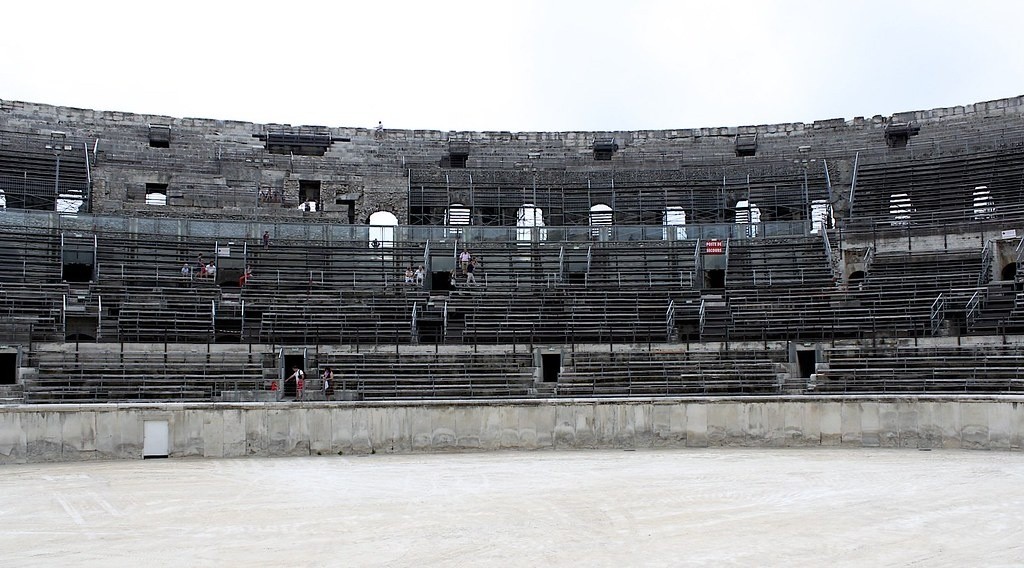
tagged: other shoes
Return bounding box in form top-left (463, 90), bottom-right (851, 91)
top-left (292, 399), bottom-right (297, 402)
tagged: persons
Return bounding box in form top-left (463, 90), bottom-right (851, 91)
top-left (239, 264), bottom-right (254, 287)
top-left (459, 248), bottom-right (482, 287)
top-left (322, 367), bottom-right (334, 401)
top-left (262, 230), bottom-right (270, 249)
top-left (305, 196), bottom-right (319, 211)
top-left (0, 195), bottom-right (6, 210)
top-left (285, 365), bottom-right (306, 402)
top-left (405, 266), bottom-right (425, 287)
top-left (374, 121), bottom-right (385, 140)
top-left (371, 239), bottom-right (381, 248)
top-left (271, 380), bottom-right (279, 392)
top-left (181, 252), bottom-right (215, 282)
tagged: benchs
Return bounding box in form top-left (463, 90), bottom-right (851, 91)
top-left (0, 121), bottom-right (1024, 410)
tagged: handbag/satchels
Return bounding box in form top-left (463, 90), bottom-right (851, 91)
top-left (324, 381), bottom-right (329, 390)
